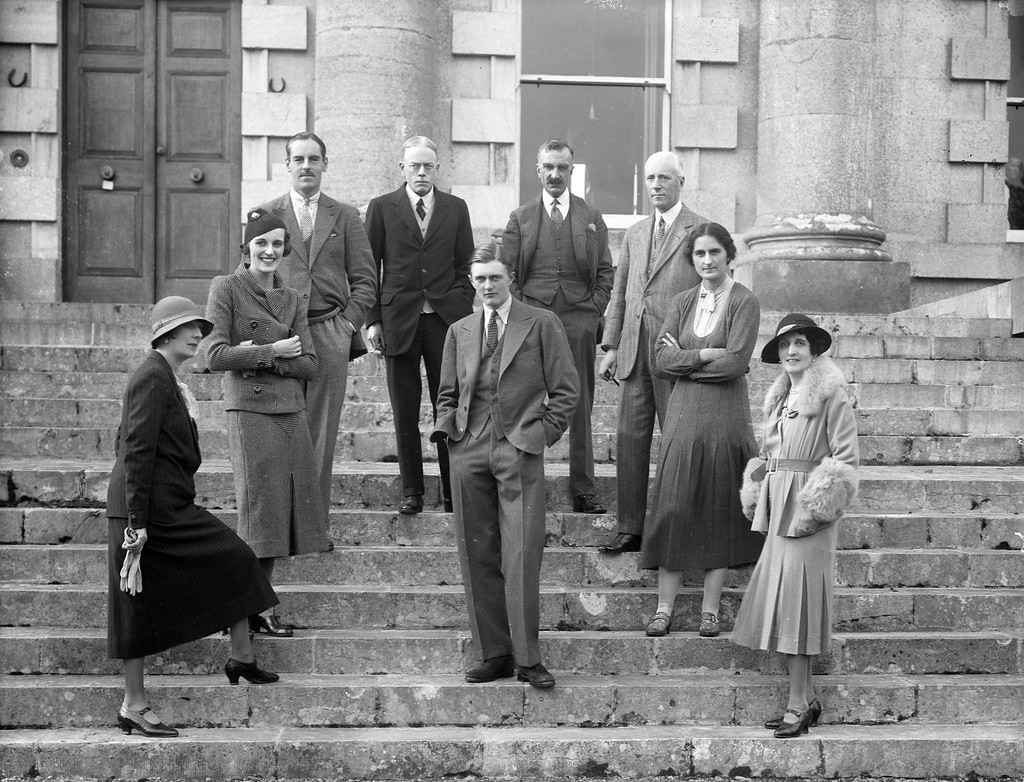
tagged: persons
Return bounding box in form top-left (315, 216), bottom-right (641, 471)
top-left (259, 132), bottom-right (378, 553)
top-left (365, 136), bottom-right (475, 513)
top-left (741, 312), bottom-right (860, 740)
top-left (597, 150), bottom-right (713, 554)
top-left (436, 244), bottom-right (580, 688)
top-left (205, 208), bottom-right (329, 638)
top-left (504, 139), bottom-right (614, 514)
top-left (648, 221), bottom-right (761, 638)
top-left (106, 295), bottom-right (279, 737)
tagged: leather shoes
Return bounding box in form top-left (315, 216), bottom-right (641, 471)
top-left (444, 499), bottom-right (453, 512)
top-left (398, 494), bottom-right (424, 514)
top-left (597, 532), bottom-right (642, 554)
top-left (574, 494), bottom-right (607, 514)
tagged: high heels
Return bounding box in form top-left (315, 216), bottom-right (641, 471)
top-left (765, 696), bottom-right (822, 729)
top-left (225, 657), bottom-right (279, 685)
top-left (117, 705), bottom-right (179, 737)
top-left (248, 612), bottom-right (294, 636)
top-left (646, 608), bottom-right (674, 636)
top-left (698, 612), bottom-right (721, 636)
top-left (774, 708), bottom-right (812, 736)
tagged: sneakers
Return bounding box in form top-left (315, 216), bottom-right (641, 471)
top-left (465, 653), bottom-right (515, 682)
top-left (518, 662), bottom-right (555, 688)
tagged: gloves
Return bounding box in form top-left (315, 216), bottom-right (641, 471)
top-left (118, 526), bottom-right (144, 595)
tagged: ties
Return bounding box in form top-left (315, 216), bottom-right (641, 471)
top-left (488, 310), bottom-right (499, 353)
top-left (551, 198), bottom-right (564, 231)
top-left (416, 199), bottom-right (426, 221)
top-left (301, 198), bottom-right (314, 242)
top-left (654, 215), bottom-right (665, 249)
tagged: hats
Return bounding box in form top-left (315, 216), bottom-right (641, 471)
top-left (761, 313), bottom-right (832, 363)
top-left (244, 208), bottom-right (286, 246)
top-left (150, 296), bottom-right (214, 347)
